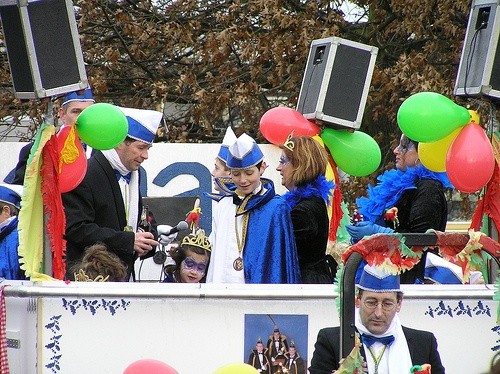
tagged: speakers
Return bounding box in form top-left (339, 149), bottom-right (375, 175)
top-left (296, 37), bottom-right (379, 132)
top-left (1, 0), bottom-right (89, 100)
top-left (452, 1), bottom-right (500, 100)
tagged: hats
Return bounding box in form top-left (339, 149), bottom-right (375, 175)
top-left (357, 261), bottom-right (401, 293)
top-left (218, 126), bottom-right (237, 163)
top-left (0, 179), bottom-right (23, 210)
top-left (227, 132), bottom-right (265, 170)
top-left (61, 82), bottom-right (96, 107)
top-left (117, 106), bottom-right (164, 145)
top-left (425, 251), bottom-right (463, 284)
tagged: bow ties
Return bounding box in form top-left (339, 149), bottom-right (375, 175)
top-left (362, 333), bottom-right (395, 347)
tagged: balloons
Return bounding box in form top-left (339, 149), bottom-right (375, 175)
top-left (304, 133), bottom-right (336, 201)
top-left (396, 92), bottom-right (470, 144)
top-left (74, 102), bottom-right (129, 150)
top-left (446, 119), bottom-right (496, 194)
top-left (47, 123), bottom-right (87, 194)
top-left (259, 107), bottom-right (322, 147)
top-left (320, 127), bottom-right (382, 177)
top-left (418, 110), bottom-right (480, 173)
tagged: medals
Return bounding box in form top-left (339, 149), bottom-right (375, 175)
top-left (123, 224), bottom-right (134, 234)
top-left (232, 256), bottom-right (244, 270)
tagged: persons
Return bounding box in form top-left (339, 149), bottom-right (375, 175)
top-left (61, 244), bottom-right (128, 286)
top-left (1, 177), bottom-right (43, 283)
top-left (273, 129), bottom-right (336, 284)
top-left (13, 82), bottom-right (101, 191)
top-left (305, 263), bottom-right (446, 374)
top-left (344, 130), bottom-right (449, 285)
top-left (203, 124), bottom-right (245, 284)
top-left (56, 110), bottom-right (159, 283)
top-left (159, 231), bottom-right (213, 285)
top-left (247, 324), bottom-right (305, 374)
top-left (212, 133), bottom-right (301, 284)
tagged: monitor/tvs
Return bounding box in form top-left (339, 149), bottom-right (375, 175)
top-left (143, 196), bottom-right (199, 240)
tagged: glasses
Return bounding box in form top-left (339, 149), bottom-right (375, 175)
top-left (357, 297), bottom-right (399, 311)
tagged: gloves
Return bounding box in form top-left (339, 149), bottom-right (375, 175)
top-left (157, 225), bottom-right (178, 246)
top-left (344, 221), bottom-right (393, 242)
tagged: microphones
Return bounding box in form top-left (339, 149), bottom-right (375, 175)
top-left (157, 220), bottom-right (188, 237)
